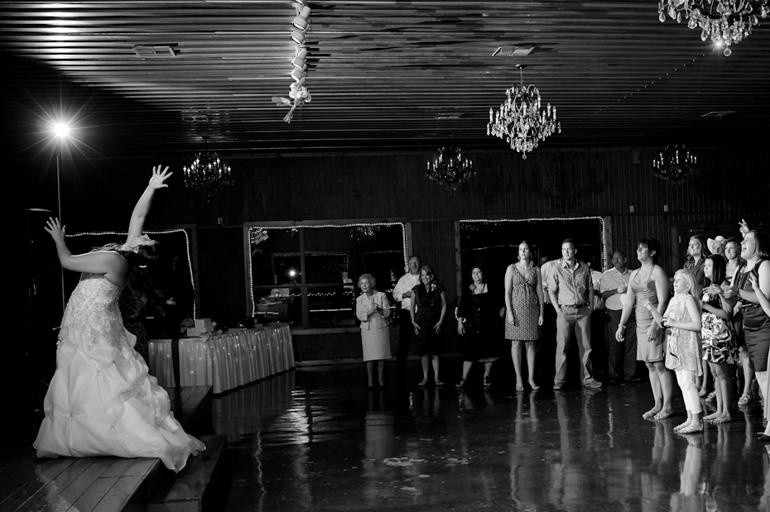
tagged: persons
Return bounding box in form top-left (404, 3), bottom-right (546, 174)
top-left (356, 255), bottom-right (447, 387)
top-left (32, 163), bottom-right (208, 474)
top-left (454, 219), bottom-right (770, 442)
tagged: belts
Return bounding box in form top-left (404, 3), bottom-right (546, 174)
top-left (559, 304), bottom-right (589, 308)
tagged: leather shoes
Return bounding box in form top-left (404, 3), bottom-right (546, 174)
top-left (516, 379), bottom-right (524, 392)
top-left (527, 382), bottom-right (539, 390)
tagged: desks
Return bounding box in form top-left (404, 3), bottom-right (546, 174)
top-left (148, 323), bottom-right (296, 394)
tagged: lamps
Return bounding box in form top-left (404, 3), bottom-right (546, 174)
top-left (424, 132), bottom-right (477, 196)
top-left (181, 133), bottom-right (235, 203)
top-left (488, 70), bottom-right (561, 160)
top-left (651, 132), bottom-right (704, 184)
top-left (289, 0), bottom-right (311, 98)
top-left (658, 0), bottom-right (769, 57)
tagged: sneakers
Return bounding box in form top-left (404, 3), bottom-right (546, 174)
top-left (642, 408), bottom-right (674, 419)
top-left (455, 378), bottom-right (466, 387)
top-left (435, 379), bottom-right (445, 387)
top-left (553, 385), bottom-right (563, 390)
top-left (699, 387), bottom-right (731, 424)
top-left (673, 420), bottom-right (704, 434)
top-left (418, 380), bottom-right (426, 386)
top-left (380, 385), bottom-right (385, 389)
top-left (483, 377), bottom-right (491, 386)
top-left (582, 378), bottom-right (601, 389)
top-left (738, 391), bottom-right (753, 404)
top-left (367, 386), bottom-right (374, 391)
